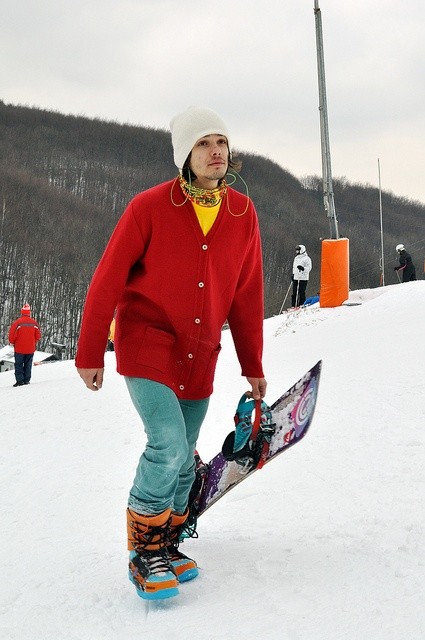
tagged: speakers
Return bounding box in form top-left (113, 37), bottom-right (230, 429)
top-left (170, 507), bottom-right (199, 582)
top-left (127, 508), bottom-right (180, 599)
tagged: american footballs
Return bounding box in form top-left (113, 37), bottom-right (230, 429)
top-left (183, 359), bottom-right (322, 517)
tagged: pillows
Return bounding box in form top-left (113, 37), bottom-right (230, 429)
top-left (395, 244), bottom-right (405, 254)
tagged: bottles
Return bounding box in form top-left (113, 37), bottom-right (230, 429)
top-left (170, 106), bottom-right (232, 177)
top-left (21, 303), bottom-right (31, 314)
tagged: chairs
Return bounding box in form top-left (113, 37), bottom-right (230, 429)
top-left (24, 379), bottom-right (30, 384)
top-left (13, 380), bottom-right (24, 387)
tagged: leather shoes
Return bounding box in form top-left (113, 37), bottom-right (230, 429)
top-left (291, 274), bottom-right (294, 281)
top-left (297, 265), bottom-right (304, 271)
top-left (394, 267), bottom-right (399, 271)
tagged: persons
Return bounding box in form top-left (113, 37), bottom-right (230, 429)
top-left (394, 243), bottom-right (417, 282)
top-left (106, 308), bottom-right (117, 352)
top-left (75, 107), bottom-right (269, 601)
top-left (291, 244), bottom-right (312, 309)
top-left (8, 304), bottom-right (41, 387)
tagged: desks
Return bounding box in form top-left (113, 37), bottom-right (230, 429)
top-left (371, 133), bottom-right (389, 287)
